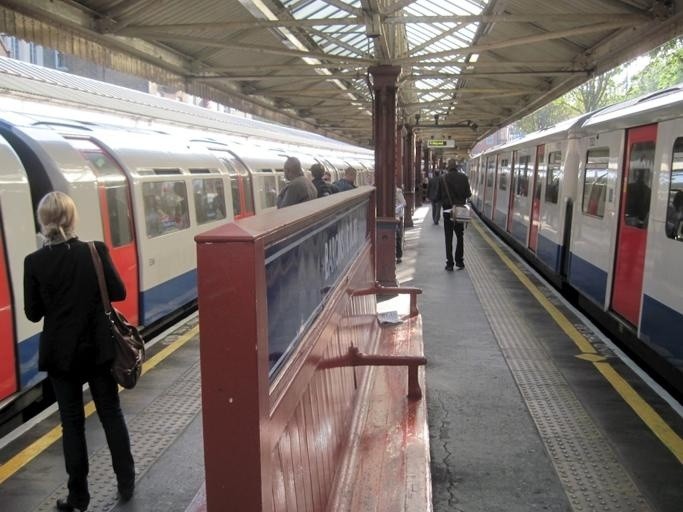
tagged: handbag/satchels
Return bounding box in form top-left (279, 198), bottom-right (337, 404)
top-left (450, 203), bottom-right (472, 222)
top-left (89, 241), bottom-right (146, 389)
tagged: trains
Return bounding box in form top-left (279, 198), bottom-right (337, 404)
top-left (466, 82), bottom-right (683, 393)
top-left (1, 95), bottom-right (375, 427)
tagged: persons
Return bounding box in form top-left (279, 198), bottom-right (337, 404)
top-left (274, 157), bottom-right (358, 209)
top-left (173, 182), bottom-right (190, 228)
top-left (395, 185), bottom-right (407, 263)
top-left (23, 190), bottom-right (135, 512)
top-left (436, 158), bottom-right (471, 270)
top-left (211, 180), bottom-right (227, 219)
top-left (623, 167), bottom-right (651, 228)
top-left (427, 168), bottom-right (443, 224)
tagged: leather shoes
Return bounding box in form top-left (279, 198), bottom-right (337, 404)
top-left (444, 266), bottom-right (453, 271)
top-left (118, 481), bottom-right (135, 501)
top-left (456, 261), bottom-right (464, 267)
top-left (56, 496), bottom-right (88, 512)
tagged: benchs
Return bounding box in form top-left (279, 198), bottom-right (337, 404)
top-left (270, 242), bottom-right (434, 511)
